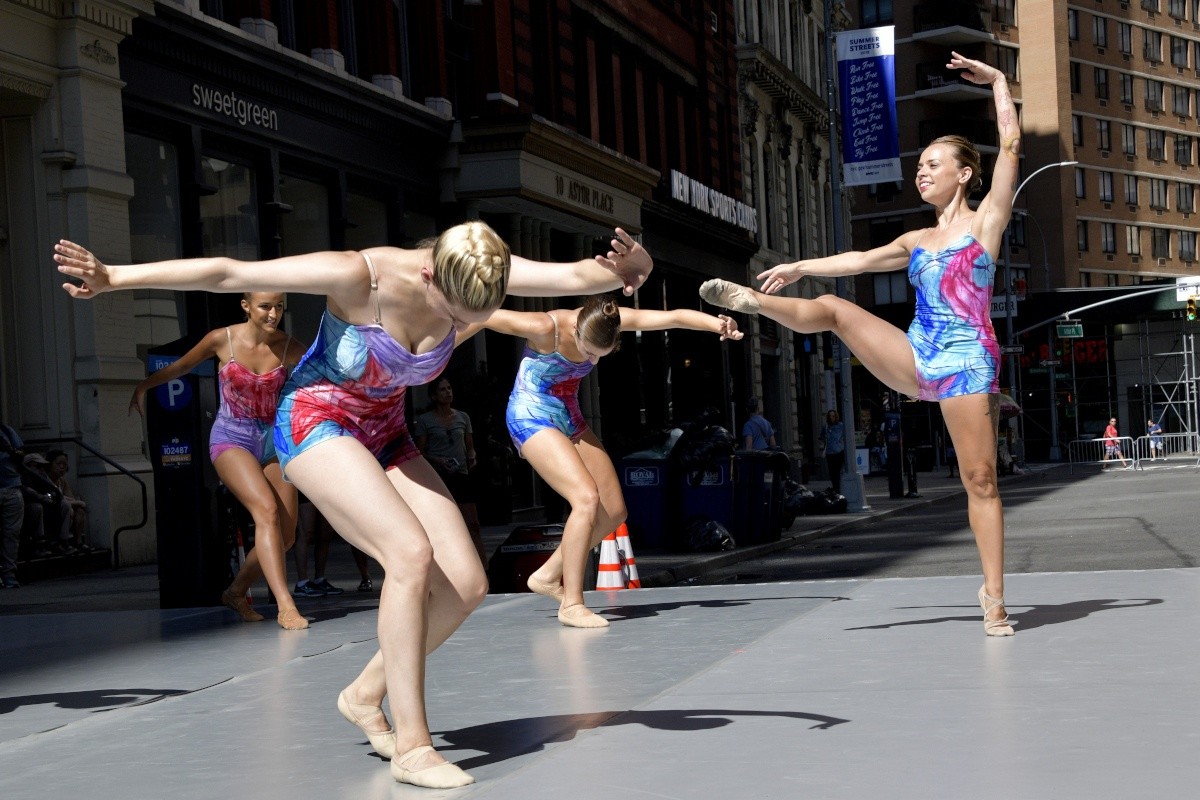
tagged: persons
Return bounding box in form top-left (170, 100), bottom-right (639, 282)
top-left (1147, 420), bottom-right (1164, 458)
top-left (53, 221), bottom-right (654, 790)
top-left (292, 375), bottom-right (489, 598)
top-left (819, 409), bottom-right (846, 492)
top-left (0, 413), bottom-right (95, 589)
top-left (698, 51), bottom-right (1022, 636)
top-left (451, 303), bottom-right (745, 629)
top-left (1100, 418), bottom-right (1125, 468)
top-left (943, 426), bottom-right (960, 478)
top-left (126, 291), bottom-right (312, 631)
top-left (742, 397), bottom-right (776, 453)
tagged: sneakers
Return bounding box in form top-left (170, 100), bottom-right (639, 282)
top-left (293, 580), bottom-right (326, 596)
top-left (313, 578), bottom-right (344, 594)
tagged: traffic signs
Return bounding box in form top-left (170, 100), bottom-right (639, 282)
top-left (1039, 358), bottom-right (1065, 368)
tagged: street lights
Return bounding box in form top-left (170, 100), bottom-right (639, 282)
top-left (1004, 161), bottom-right (1080, 476)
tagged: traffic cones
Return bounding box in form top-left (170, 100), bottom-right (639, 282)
top-left (594, 531), bottom-right (626, 590)
top-left (615, 521), bottom-right (642, 590)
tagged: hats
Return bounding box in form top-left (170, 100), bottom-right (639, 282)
top-left (22, 452), bottom-right (51, 464)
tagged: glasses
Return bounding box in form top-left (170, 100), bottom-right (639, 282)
top-left (446, 429), bottom-right (454, 445)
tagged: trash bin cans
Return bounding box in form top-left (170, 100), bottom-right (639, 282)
top-left (488, 524), bottom-right (595, 593)
top-left (622, 447), bottom-right (792, 536)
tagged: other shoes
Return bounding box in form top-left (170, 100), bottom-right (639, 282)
top-left (31, 546), bottom-right (53, 558)
top-left (2, 572), bottom-right (20, 588)
top-left (358, 578), bottom-right (373, 592)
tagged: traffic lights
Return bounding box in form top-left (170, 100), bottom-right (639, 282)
top-left (1186, 299), bottom-right (1196, 321)
top-left (1055, 335), bottom-right (1064, 357)
top-left (1065, 391), bottom-right (1076, 418)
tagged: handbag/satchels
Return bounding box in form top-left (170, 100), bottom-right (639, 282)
top-left (812, 423), bottom-right (828, 459)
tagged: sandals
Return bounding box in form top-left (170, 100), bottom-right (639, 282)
top-left (58, 543), bottom-right (79, 554)
top-left (74, 542), bottom-right (95, 552)
